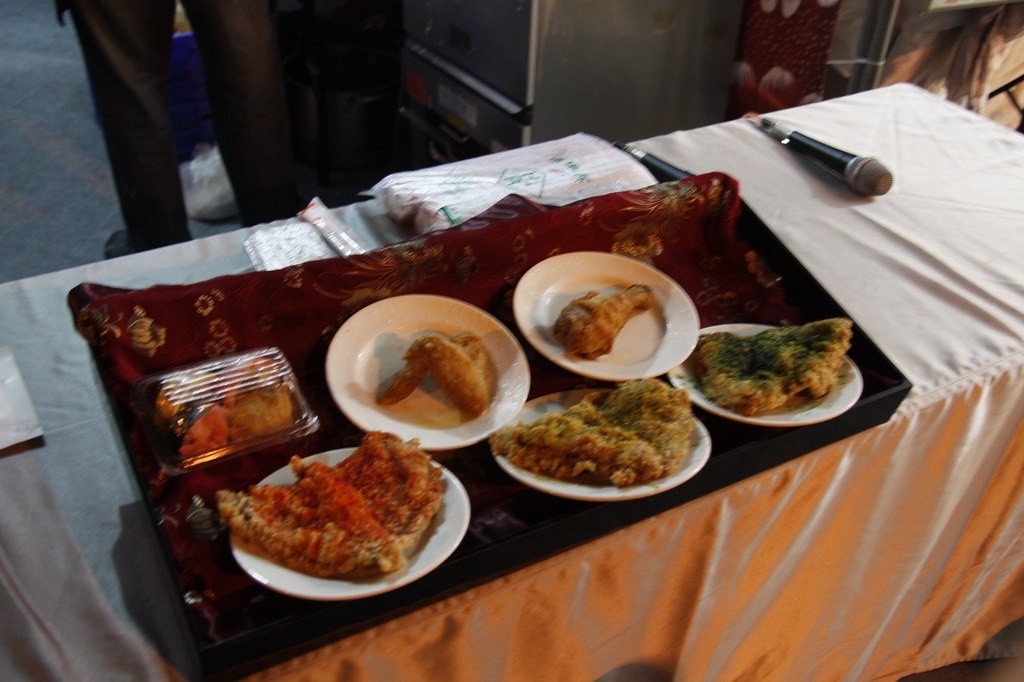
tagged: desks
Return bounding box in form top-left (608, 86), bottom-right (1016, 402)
top-left (0, 81), bottom-right (1024, 682)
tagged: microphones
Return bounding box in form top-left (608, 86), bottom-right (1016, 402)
top-left (759, 118), bottom-right (893, 196)
top-left (613, 141), bottom-right (697, 184)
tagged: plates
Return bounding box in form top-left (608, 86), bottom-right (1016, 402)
top-left (491, 389), bottom-right (712, 503)
top-left (229, 447), bottom-right (470, 601)
top-left (512, 251), bottom-right (700, 381)
top-left (324, 295), bottom-right (531, 450)
top-left (667, 324), bottom-right (863, 427)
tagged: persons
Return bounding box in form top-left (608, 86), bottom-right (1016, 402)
top-left (53, 0), bottom-right (299, 252)
